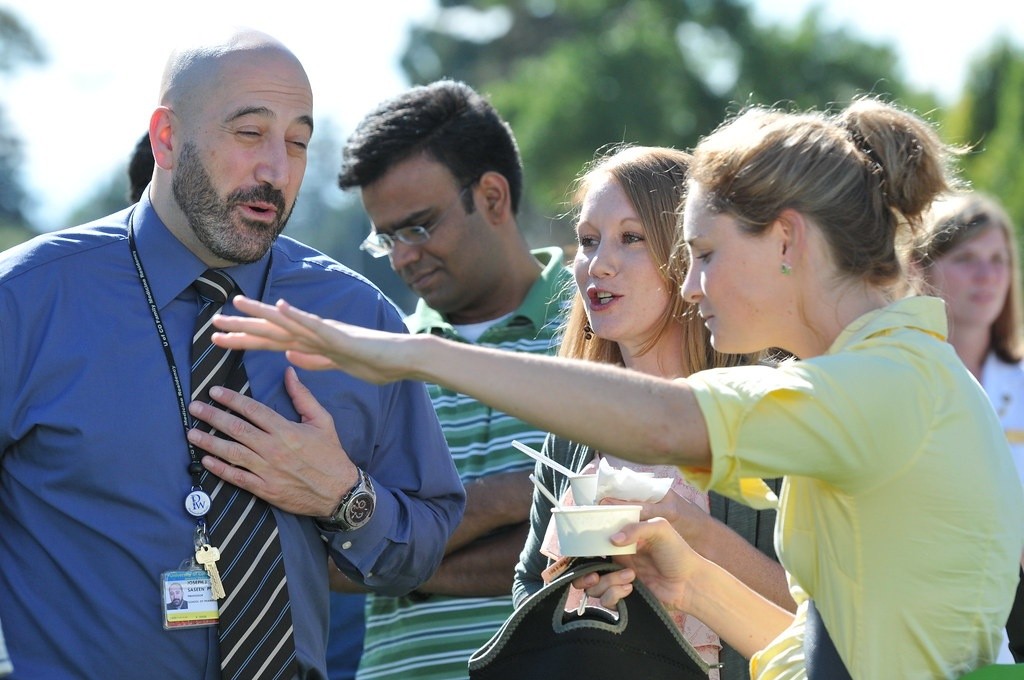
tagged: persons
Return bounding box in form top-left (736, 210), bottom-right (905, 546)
top-left (209, 95), bottom-right (1023, 680)
top-left (901, 189), bottom-right (1023, 466)
top-left (167, 582), bottom-right (188, 610)
top-left (336, 79), bottom-right (583, 680)
top-left (512, 146), bottom-right (795, 680)
top-left (0, 24), bottom-right (468, 680)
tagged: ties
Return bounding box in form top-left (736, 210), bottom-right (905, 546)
top-left (188, 267), bottom-right (300, 680)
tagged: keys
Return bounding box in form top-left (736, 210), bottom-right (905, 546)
top-left (195, 528), bottom-right (226, 602)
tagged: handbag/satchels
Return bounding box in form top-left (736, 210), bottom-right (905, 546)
top-left (467, 556), bottom-right (714, 680)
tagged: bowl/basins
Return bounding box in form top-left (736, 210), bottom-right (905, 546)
top-left (567, 472), bottom-right (656, 505)
top-left (551, 505), bottom-right (643, 557)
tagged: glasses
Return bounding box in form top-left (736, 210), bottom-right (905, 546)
top-left (358, 176), bottom-right (481, 259)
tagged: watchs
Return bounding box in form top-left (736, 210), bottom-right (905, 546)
top-left (316, 467), bottom-right (377, 533)
